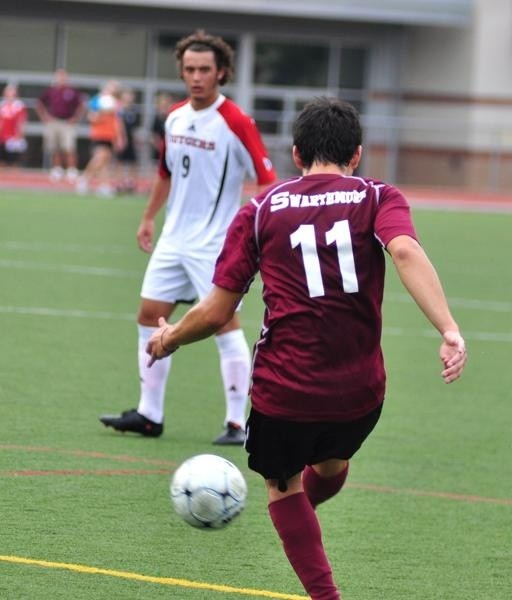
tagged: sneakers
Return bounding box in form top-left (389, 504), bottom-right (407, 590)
top-left (212, 429), bottom-right (247, 446)
top-left (98, 408), bottom-right (165, 438)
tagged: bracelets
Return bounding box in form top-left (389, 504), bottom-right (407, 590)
top-left (160, 327), bottom-right (179, 353)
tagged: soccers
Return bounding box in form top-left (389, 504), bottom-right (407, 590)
top-left (172, 455), bottom-right (246, 531)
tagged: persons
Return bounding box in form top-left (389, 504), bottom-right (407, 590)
top-left (97, 29), bottom-right (276, 446)
top-left (145, 96), bottom-right (468, 600)
top-left (149, 89), bottom-right (176, 174)
top-left (113, 85), bottom-right (143, 192)
top-left (0, 78), bottom-right (27, 166)
top-left (76, 78), bottom-right (123, 198)
top-left (35, 68), bottom-right (84, 180)
